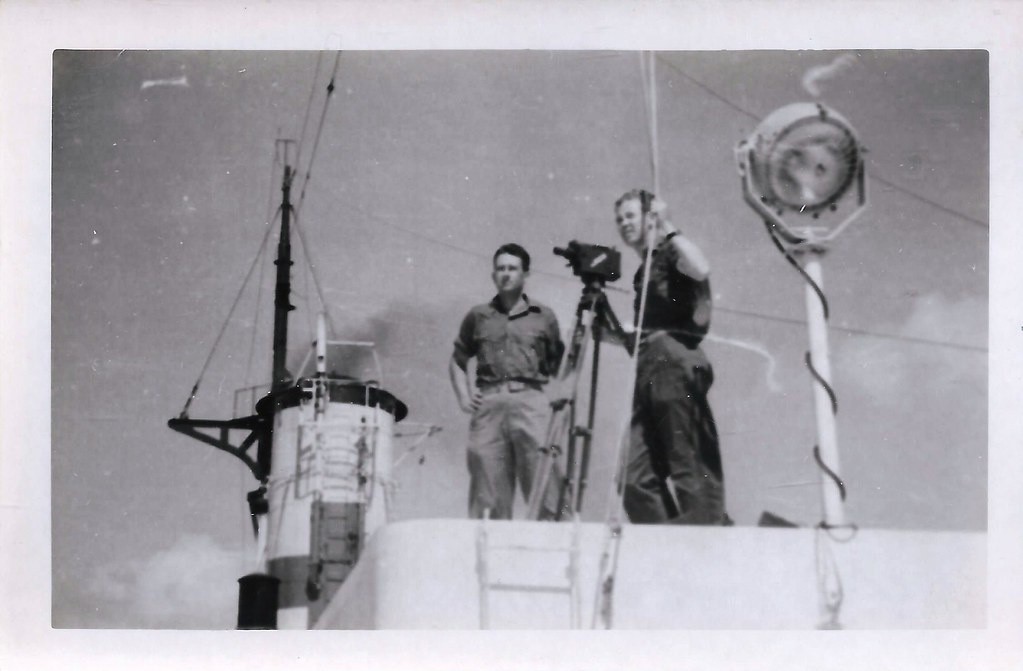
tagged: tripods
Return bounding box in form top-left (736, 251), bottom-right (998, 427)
top-left (522, 278), bottom-right (685, 524)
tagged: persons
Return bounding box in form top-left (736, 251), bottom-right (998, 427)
top-left (594, 187), bottom-right (727, 529)
top-left (443, 244), bottom-right (565, 518)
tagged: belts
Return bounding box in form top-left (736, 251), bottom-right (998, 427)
top-left (479, 379), bottom-right (542, 393)
